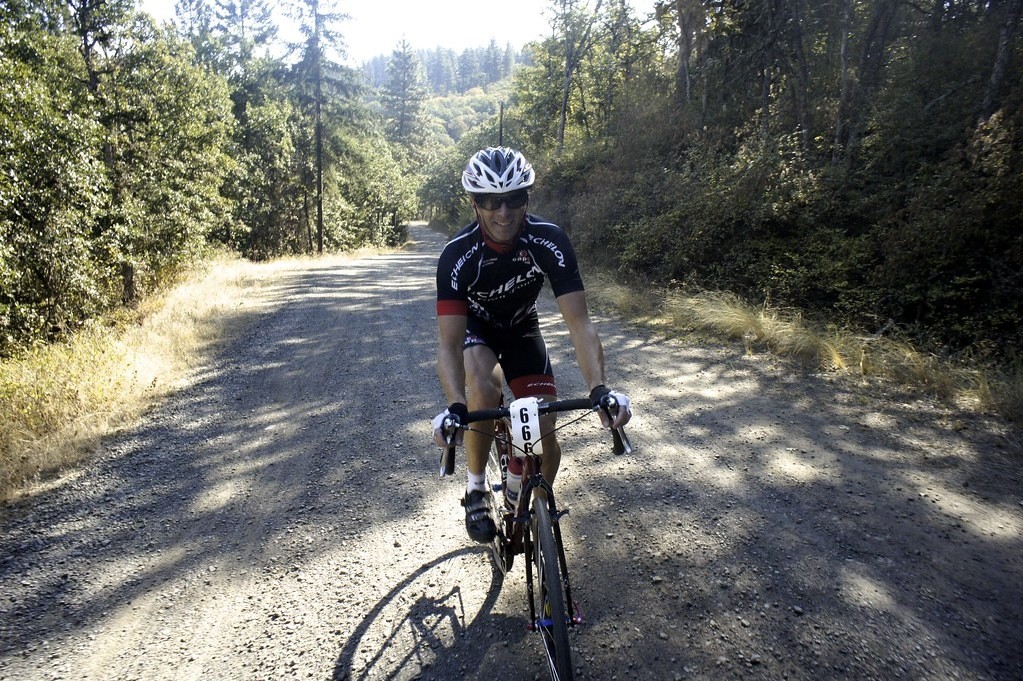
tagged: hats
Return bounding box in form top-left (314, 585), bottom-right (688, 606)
top-left (461, 145), bottom-right (536, 194)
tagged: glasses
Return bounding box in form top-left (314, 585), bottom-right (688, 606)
top-left (471, 194), bottom-right (529, 210)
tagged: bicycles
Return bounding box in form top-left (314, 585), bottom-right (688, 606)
top-left (440, 387), bottom-right (633, 681)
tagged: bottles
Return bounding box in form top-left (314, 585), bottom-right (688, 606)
top-left (506, 454), bottom-right (525, 506)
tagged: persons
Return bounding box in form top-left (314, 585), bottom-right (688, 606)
top-left (434, 145), bottom-right (632, 544)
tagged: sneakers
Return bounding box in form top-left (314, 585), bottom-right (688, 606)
top-left (462, 491), bottom-right (497, 543)
